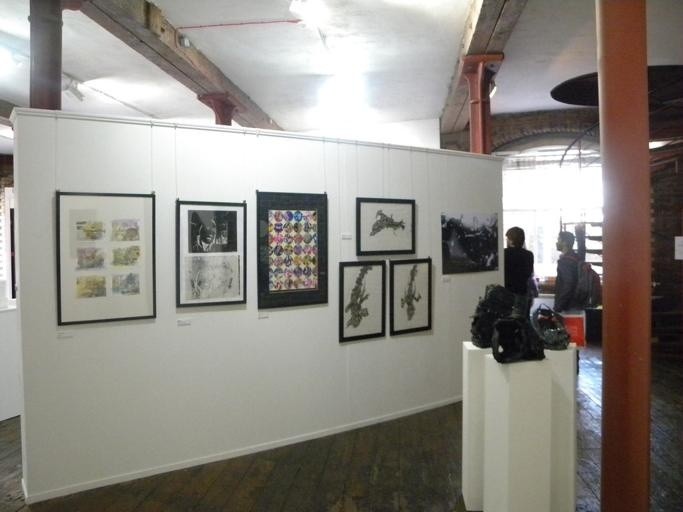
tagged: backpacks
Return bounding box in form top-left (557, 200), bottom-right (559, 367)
top-left (561, 255), bottom-right (602, 309)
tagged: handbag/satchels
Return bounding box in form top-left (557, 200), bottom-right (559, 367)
top-left (526, 277), bottom-right (539, 298)
top-left (470, 284), bottom-right (570, 364)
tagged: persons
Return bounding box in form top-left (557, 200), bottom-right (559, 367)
top-left (503, 226), bottom-right (533, 317)
top-left (553, 231), bottom-right (581, 313)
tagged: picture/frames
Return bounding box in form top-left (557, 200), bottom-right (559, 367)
top-left (339, 260), bottom-right (387, 343)
top-left (56, 191), bottom-right (156, 326)
top-left (257, 192), bottom-right (328, 308)
top-left (175, 199), bottom-right (247, 307)
top-left (389, 258), bottom-right (432, 336)
top-left (355, 197), bottom-right (416, 256)
